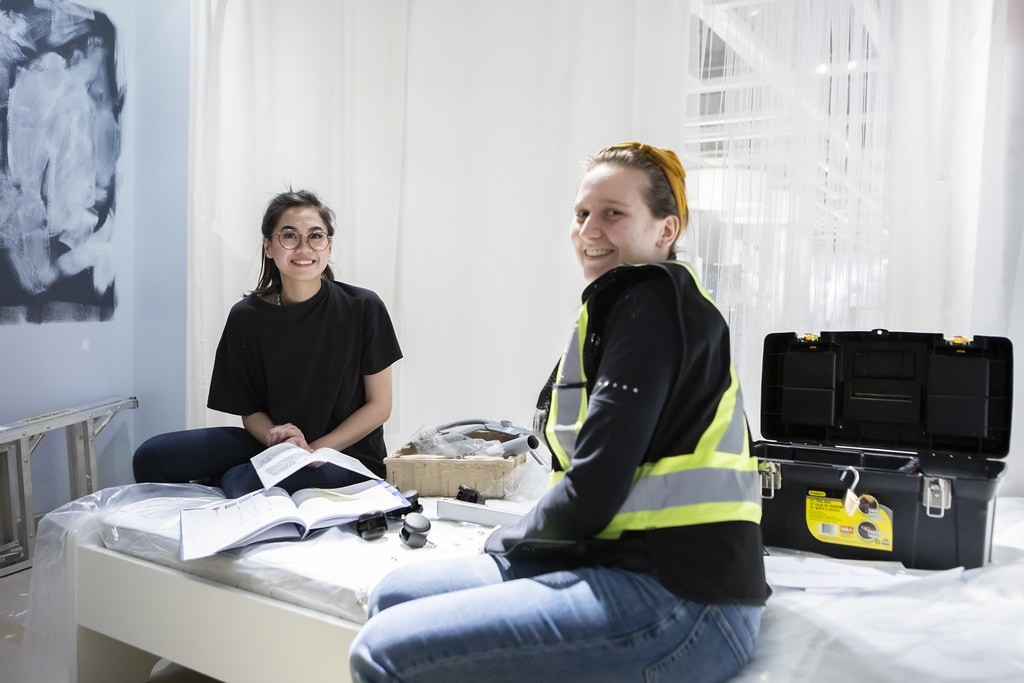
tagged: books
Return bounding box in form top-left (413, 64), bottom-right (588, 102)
top-left (179, 475), bottom-right (411, 562)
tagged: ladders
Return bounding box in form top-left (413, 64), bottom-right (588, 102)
top-left (1, 397), bottom-right (138, 578)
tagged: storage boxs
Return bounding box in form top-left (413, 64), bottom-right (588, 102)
top-left (381, 424), bottom-right (527, 500)
top-left (750, 328), bottom-right (1015, 571)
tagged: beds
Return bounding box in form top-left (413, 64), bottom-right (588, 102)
top-left (64, 497), bottom-right (1024, 683)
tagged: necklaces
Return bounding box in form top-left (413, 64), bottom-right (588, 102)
top-left (275, 290), bottom-right (282, 306)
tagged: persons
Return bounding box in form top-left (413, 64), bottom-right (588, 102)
top-left (350, 139), bottom-right (770, 683)
top-left (132, 184), bottom-right (405, 502)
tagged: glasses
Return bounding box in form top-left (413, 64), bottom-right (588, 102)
top-left (269, 229), bottom-right (333, 251)
